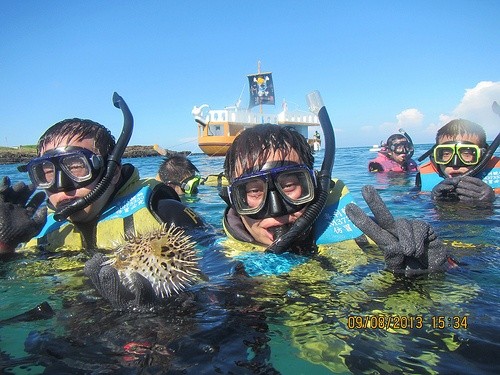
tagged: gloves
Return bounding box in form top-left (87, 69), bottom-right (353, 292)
top-left (83, 253), bottom-right (249, 312)
top-left (345, 185), bottom-right (447, 276)
top-left (431, 177), bottom-right (495, 202)
top-left (0, 177), bottom-right (47, 245)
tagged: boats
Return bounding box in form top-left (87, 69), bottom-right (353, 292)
top-left (369, 144), bottom-right (381, 153)
top-left (192, 61), bottom-right (322, 157)
top-left (154, 144), bottom-right (167, 157)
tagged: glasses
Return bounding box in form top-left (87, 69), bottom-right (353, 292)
top-left (28, 146), bottom-right (103, 193)
top-left (170, 175), bottom-right (201, 193)
top-left (388, 140), bottom-right (410, 154)
top-left (434, 145), bottom-right (487, 166)
top-left (227, 161), bottom-right (317, 220)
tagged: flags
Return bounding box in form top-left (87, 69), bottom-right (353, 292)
top-left (248, 73), bottom-right (275, 109)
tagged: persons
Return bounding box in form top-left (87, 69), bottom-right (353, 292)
top-left (0, 119), bottom-right (244, 315)
top-left (314, 130), bottom-right (320, 139)
top-left (157, 155), bottom-right (201, 197)
top-left (414, 119), bottom-right (500, 204)
top-left (84, 124), bottom-right (464, 319)
top-left (368, 134), bottom-right (418, 173)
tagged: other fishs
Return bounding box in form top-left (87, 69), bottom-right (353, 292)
top-left (99, 220), bottom-right (203, 298)
